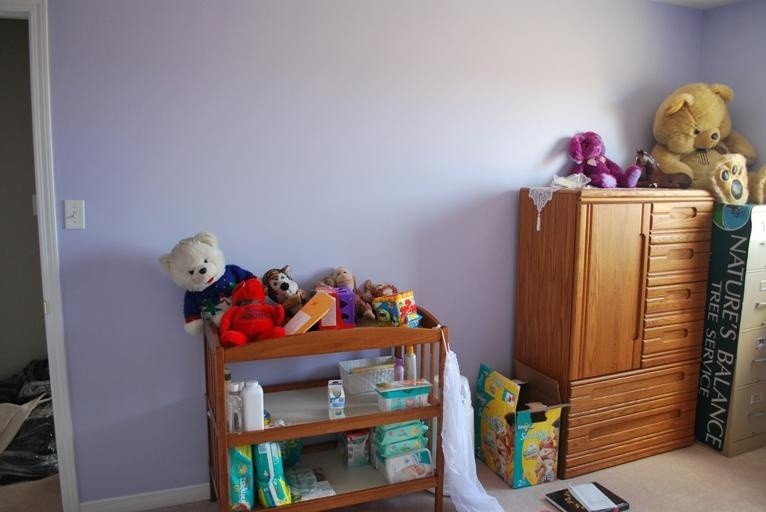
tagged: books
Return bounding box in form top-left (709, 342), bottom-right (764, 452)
top-left (544, 481), bottom-right (630, 512)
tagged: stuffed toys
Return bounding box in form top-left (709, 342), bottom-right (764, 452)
top-left (636, 147), bottom-right (693, 188)
top-left (648, 82), bottom-right (766, 207)
top-left (566, 131), bottom-right (643, 187)
top-left (157, 231), bottom-right (398, 347)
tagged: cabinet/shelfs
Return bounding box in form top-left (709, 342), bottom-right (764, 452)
top-left (202, 306), bottom-right (450, 512)
top-left (513, 188), bottom-right (714, 478)
top-left (693, 202), bottom-right (765, 459)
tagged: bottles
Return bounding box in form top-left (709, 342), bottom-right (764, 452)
top-left (394, 345), bottom-right (416, 387)
top-left (224, 366), bottom-right (264, 433)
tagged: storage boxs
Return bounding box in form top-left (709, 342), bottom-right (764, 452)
top-left (472, 362), bottom-right (575, 488)
